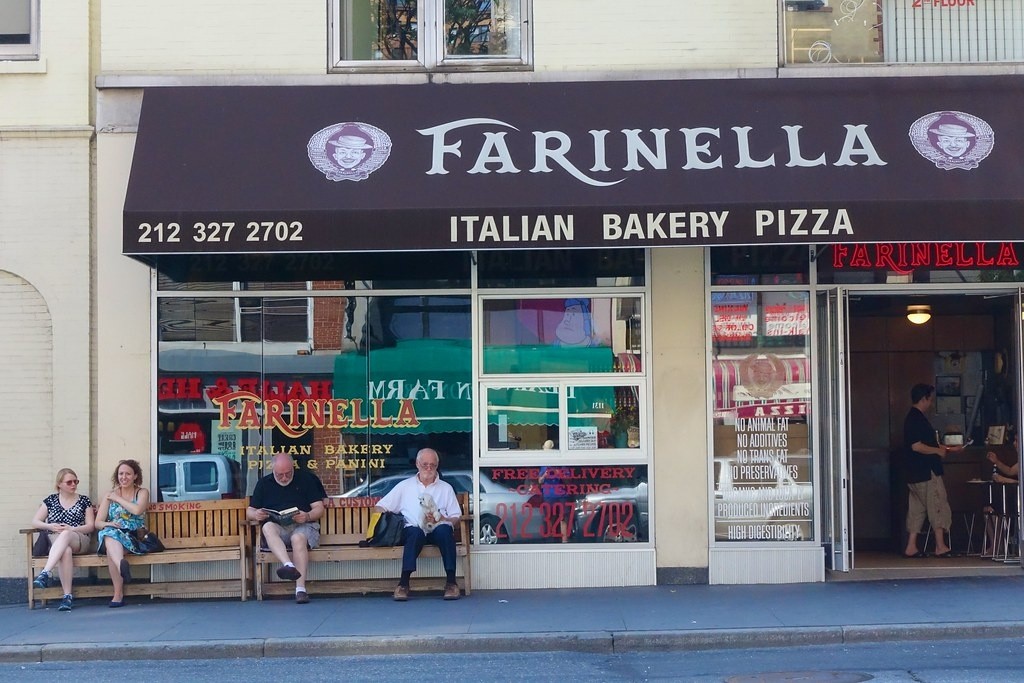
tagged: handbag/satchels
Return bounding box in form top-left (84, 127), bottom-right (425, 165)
top-left (359, 512), bottom-right (404, 547)
top-left (32, 531), bottom-right (51, 556)
top-left (133, 528), bottom-right (165, 554)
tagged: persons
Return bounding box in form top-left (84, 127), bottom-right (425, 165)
top-left (32, 467), bottom-right (94, 611)
top-left (374, 448), bottom-right (462, 601)
top-left (246, 452), bottom-right (325, 604)
top-left (538, 466), bottom-right (573, 544)
top-left (981, 435), bottom-right (1019, 556)
top-left (94, 459), bottom-right (149, 607)
top-left (902, 384), bottom-right (962, 557)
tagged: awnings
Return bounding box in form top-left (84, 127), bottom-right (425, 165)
top-left (714, 357), bottom-right (807, 419)
top-left (333, 340), bottom-right (615, 435)
top-left (121, 72), bottom-right (1024, 255)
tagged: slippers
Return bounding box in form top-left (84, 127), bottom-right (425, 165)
top-left (936, 549), bottom-right (962, 558)
top-left (905, 550), bottom-right (930, 558)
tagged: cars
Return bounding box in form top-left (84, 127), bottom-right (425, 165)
top-left (328, 470), bottom-right (548, 545)
top-left (577, 457), bottom-right (812, 543)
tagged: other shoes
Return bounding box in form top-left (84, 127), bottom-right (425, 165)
top-left (276, 565), bottom-right (301, 580)
top-left (120, 559), bottom-right (131, 584)
top-left (109, 600), bottom-right (124, 608)
top-left (296, 591), bottom-right (309, 603)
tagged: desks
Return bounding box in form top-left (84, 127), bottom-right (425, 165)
top-left (960, 478), bottom-right (1022, 562)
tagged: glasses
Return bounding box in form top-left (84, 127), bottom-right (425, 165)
top-left (62, 480), bottom-right (79, 485)
top-left (418, 461), bottom-right (438, 470)
top-left (274, 467), bottom-right (292, 478)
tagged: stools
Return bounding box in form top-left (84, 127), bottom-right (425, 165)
top-left (955, 507), bottom-right (1022, 563)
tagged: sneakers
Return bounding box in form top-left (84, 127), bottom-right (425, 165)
top-left (59, 594), bottom-right (72, 611)
top-left (444, 582), bottom-right (460, 600)
top-left (33, 570), bottom-right (49, 588)
top-left (394, 585), bottom-right (409, 600)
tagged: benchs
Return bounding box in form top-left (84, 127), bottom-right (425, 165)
top-left (17, 497), bottom-right (252, 601)
top-left (252, 492), bottom-right (471, 602)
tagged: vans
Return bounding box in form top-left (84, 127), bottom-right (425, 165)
top-left (158, 454), bottom-right (234, 502)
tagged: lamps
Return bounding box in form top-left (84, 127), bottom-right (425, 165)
top-left (296, 342), bottom-right (312, 355)
top-left (906, 303), bottom-right (932, 325)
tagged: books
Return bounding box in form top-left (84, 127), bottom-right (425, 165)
top-left (262, 507), bottom-right (300, 527)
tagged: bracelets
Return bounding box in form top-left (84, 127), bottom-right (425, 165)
top-left (945, 446), bottom-right (950, 451)
